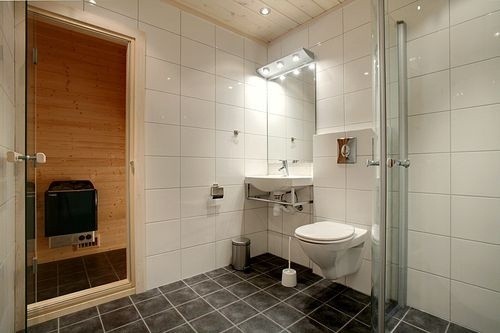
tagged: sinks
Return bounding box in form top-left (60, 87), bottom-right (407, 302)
top-left (245, 174), bottom-right (312, 195)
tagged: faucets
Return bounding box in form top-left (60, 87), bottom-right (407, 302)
top-left (292, 160), bottom-right (300, 163)
top-left (277, 159), bottom-right (291, 176)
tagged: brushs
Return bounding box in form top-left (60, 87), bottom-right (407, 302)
top-left (281, 236), bottom-right (297, 288)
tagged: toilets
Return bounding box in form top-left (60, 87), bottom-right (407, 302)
top-left (293, 220), bottom-right (369, 281)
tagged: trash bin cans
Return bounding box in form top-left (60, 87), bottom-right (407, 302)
top-left (231, 237), bottom-right (251, 271)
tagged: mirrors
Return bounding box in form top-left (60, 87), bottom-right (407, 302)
top-left (266, 62), bottom-right (317, 161)
